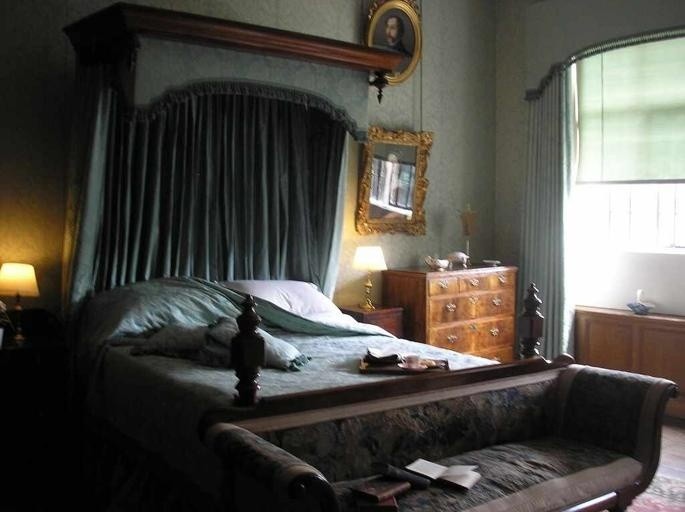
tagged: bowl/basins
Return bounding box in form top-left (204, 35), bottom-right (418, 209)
top-left (628, 302), bottom-right (655, 316)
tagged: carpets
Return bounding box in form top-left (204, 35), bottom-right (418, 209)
top-left (615, 470), bottom-right (685, 510)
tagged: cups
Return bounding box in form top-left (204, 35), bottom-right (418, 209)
top-left (425, 253), bottom-right (449, 270)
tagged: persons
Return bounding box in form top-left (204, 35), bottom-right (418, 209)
top-left (381, 15), bottom-right (414, 76)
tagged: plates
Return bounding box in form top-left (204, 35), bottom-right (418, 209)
top-left (482, 259), bottom-right (501, 267)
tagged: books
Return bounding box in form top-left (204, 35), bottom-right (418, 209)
top-left (405, 458), bottom-right (480, 491)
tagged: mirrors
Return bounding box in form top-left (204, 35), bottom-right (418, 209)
top-left (352, 123), bottom-right (437, 237)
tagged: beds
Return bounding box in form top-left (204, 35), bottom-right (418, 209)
top-left (73, 283), bottom-right (576, 512)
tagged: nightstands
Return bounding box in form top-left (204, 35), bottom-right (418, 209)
top-left (339, 302), bottom-right (405, 337)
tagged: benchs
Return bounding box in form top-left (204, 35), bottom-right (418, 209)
top-left (202, 353), bottom-right (684, 511)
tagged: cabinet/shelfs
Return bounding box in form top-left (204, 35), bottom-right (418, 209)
top-left (381, 263), bottom-right (519, 358)
top-left (573, 304), bottom-right (685, 421)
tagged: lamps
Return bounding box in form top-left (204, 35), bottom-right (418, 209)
top-left (0, 262), bottom-right (41, 346)
top-left (352, 245), bottom-right (388, 309)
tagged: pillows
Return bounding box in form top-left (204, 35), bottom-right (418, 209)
top-left (128, 321), bottom-right (312, 374)
top-left (218, 280), bottom-right (344, 329)
top-left (83, 277), bottom-right (270, 345)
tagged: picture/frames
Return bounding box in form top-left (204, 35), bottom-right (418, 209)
top-left (361, 0), bottom-right (424, 84)
